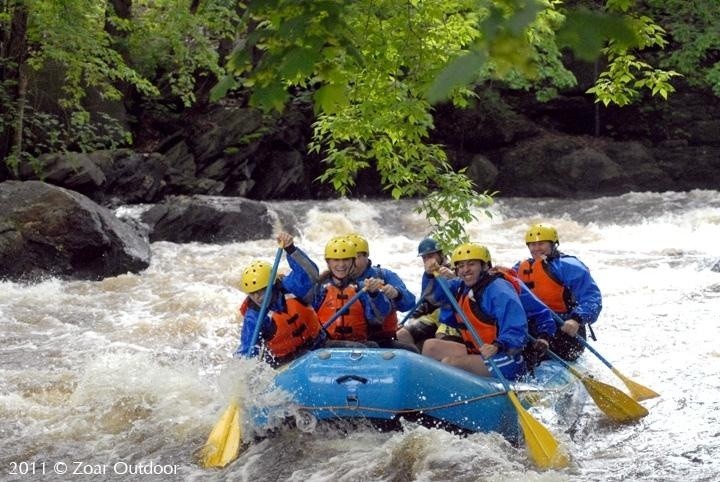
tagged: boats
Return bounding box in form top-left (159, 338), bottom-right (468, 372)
top-left (246, 348), bottom-right (596, 454)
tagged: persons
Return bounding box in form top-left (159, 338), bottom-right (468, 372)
top-left (512, 222), bottom-right (602, 360)
top-left (322, 234), bottom-right (421, 355)
top-left (312, 236), bottom-right (391, 348)
top-left (397, 239), bottom-right (464, 345)
top-left (422, 242), bottom-right (529, 380)
top-left (442, 266), bottom-right (555, 372)
top-left (233, 232), bottom-right (367, 371)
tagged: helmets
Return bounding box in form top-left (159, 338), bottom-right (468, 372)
top-left (450, 243), bottom-right (492, 270)
top-left (240, 259), bottom-right (281, 293)
top-left (418, 238), bottom-right (442, 256)
top-left (325, 237), bottom-right (358, 260)
top-left (523, 223), bottom-right (559, 245)
top-left (346, 233), bottom-right (368, 255)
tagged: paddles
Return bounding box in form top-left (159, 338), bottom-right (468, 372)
top-left (550, 309), bottom-right (659, 401)
top-left (432, 270), bottom-right (568, 470)
top-left (529, 334), bottom-right (649, 422)
top-left (201, 241), bottom-right (284, 468)
